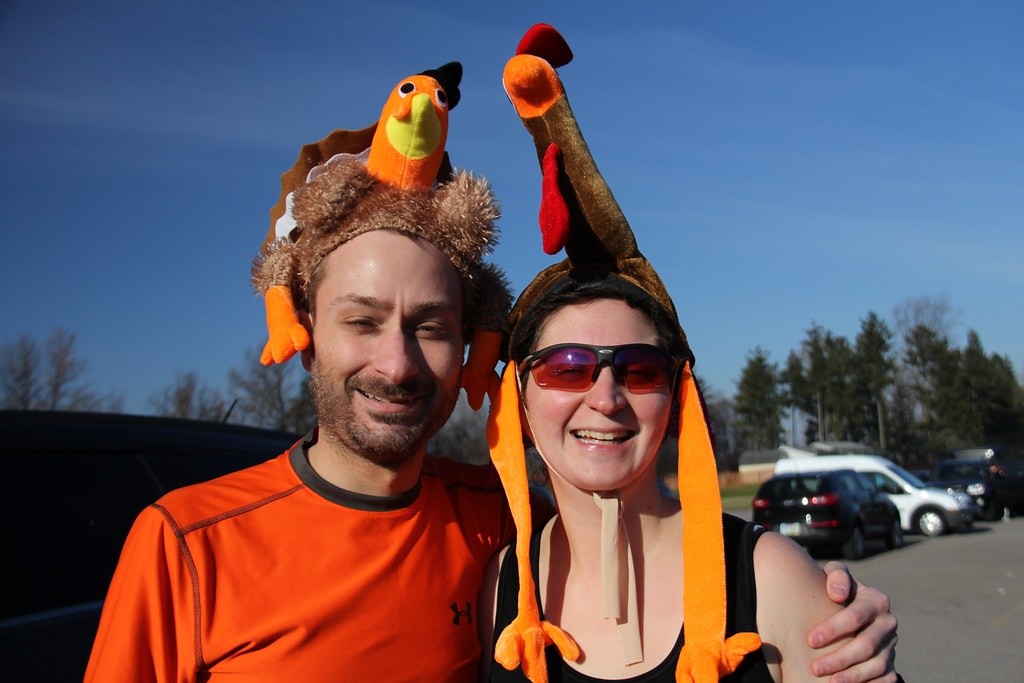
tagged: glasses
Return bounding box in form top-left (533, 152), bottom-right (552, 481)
top-left (522, 342), bottom-right (684, 392)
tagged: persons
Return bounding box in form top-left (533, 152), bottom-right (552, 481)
top-left (476, 21), bottom-right (905, 683)
top-left (83, 61), bottom-right (901, 683)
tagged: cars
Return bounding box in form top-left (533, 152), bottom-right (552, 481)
top-left (750, 469), bottom-right (907, 563)
top-left (917, 455), bottom-right (1005, 525)
top-left (775, 453), bottom-right (975, 539)
